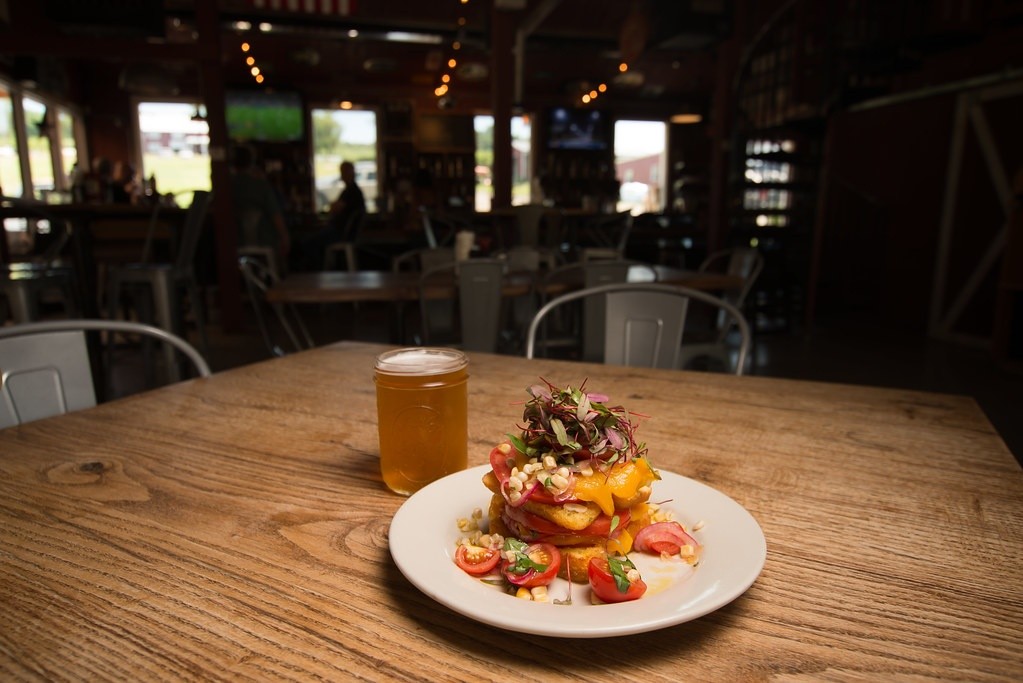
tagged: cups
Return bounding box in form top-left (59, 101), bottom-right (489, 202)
top-left (374, 347), bottom-right (469, 497)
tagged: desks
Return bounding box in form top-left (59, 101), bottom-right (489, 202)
top-left (266, 265), bottom-right (751, 355)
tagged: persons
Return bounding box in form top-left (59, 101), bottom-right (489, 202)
top-left (287, 163), bottom-right (450, 273)
top-left (70, 156), bottom-right (162, 206)
top-left (540, 164), bottom-right (712, 267)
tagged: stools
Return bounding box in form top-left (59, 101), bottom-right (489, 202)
top-left (105, 266), bottom-right (208, 385)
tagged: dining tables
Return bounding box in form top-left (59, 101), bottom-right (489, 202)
top-left (0, 340), bottom-right (1023, 683)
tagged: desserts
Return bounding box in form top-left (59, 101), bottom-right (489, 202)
top-left (455, 377), bottom-right (706, 603)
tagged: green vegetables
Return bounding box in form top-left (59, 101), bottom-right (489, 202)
top-left (607, 549), bottom-right (641, 593)
top-left (503, 378), bottom-right (644, 459)
top-left (504, 535), bottom-right (547, 573)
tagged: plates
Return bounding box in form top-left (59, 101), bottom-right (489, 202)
top-left (389, 463), bottom-right (767, 640)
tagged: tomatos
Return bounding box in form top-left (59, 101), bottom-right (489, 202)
top-left (501, 541), bottom-right (561, 585)
top-left (588, 557), bottom-right (647, 600)
top-left (457, 544), bottom-right (500, 576)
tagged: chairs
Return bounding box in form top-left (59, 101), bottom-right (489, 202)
top-left (391, 247), bottom-right (470, 349)
top-left (239, 257), bottom-right (316, 356)
top-left (417, 258), bottom-right (549, 358)
top-left (537, 260), bottom-right (691, 365)
top-left (491, 244), bottom-right (579, 358)
top-left (0, 320), bottom-right (211, 430)
top-left (679, 249), bottom-right (765, 374)
top-left (526, 283), bottom-right (751, 375)
